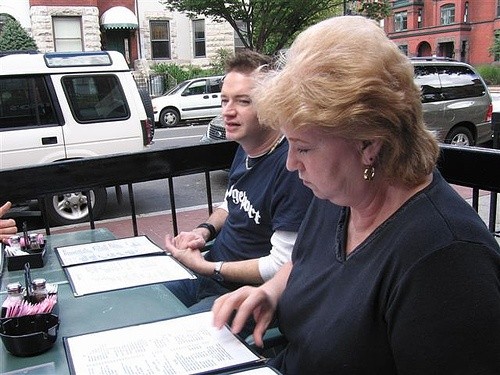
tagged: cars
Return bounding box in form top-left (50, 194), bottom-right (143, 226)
top-left (150, 75), bottom-right (223, 127)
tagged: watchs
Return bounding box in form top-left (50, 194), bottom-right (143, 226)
top-left (212, 261), bottom-right (224, 283)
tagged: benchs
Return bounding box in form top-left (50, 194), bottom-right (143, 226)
top-left (0, 140), bottom-right (240, 238)
top-left (435, 142), bottom-right (500, 242)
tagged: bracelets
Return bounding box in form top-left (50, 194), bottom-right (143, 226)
top-left (197, 223), bottom-right (216, 241)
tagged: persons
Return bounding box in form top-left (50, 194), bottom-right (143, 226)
top-left (165, 49), bottom-right (314, 314)
top-left (0, 201), bottom-right (17, 244)
top-left (211, 15), bottom-right (500, 375)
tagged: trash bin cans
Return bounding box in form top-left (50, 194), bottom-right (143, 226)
top-left (150, 73), bottom-right (165, 97)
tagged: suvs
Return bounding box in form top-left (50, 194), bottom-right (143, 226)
top-left (207, 56), bottom-right (495, 144)
top-left (0, 48), bottom-right (156, 227)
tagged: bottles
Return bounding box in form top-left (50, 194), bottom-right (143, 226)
top-left (28, 233), bottom-right (40, 249)
top-left (10, 236), bottom-right (21, 250)
top-left (6, 283), bottom-right (23, 307)
top-left (31, 278), bottom-right (47, 302)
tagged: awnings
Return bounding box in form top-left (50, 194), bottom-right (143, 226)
top-left (99, 5), bottom-right (139, 31)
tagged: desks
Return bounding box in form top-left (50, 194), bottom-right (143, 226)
top-left (0, 227), bottom-right (191, 375)
